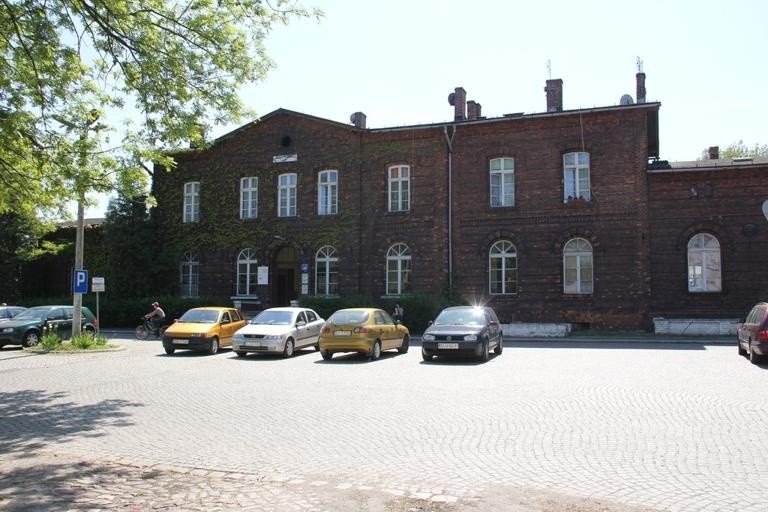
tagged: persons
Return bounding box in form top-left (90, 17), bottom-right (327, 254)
top-left (144, 302), bottom-right (166, 341)
top-left (394, 301), bottom-right (404, 322)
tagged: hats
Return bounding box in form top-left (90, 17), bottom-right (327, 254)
top-left (151, 302), bottom-right (159, 306)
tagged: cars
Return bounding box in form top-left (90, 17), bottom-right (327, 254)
top-left (736, 301), bottom-right (768, 362)
top-left (318, 307), bottom-right (410, 361)
top-left (162, 307), bottom-right (247, 355)
top-left (420, 305), bottom-right (503, 361)
top-left (232, 306), bottom-right (325, 357)
top-left (0, 305), bottom-right (97, 348)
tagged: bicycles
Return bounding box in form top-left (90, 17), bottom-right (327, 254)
top-left (135, 317), bottom-right (169, 340)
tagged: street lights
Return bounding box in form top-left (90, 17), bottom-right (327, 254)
top-left (52, 108), bottom-right (106, 345)
